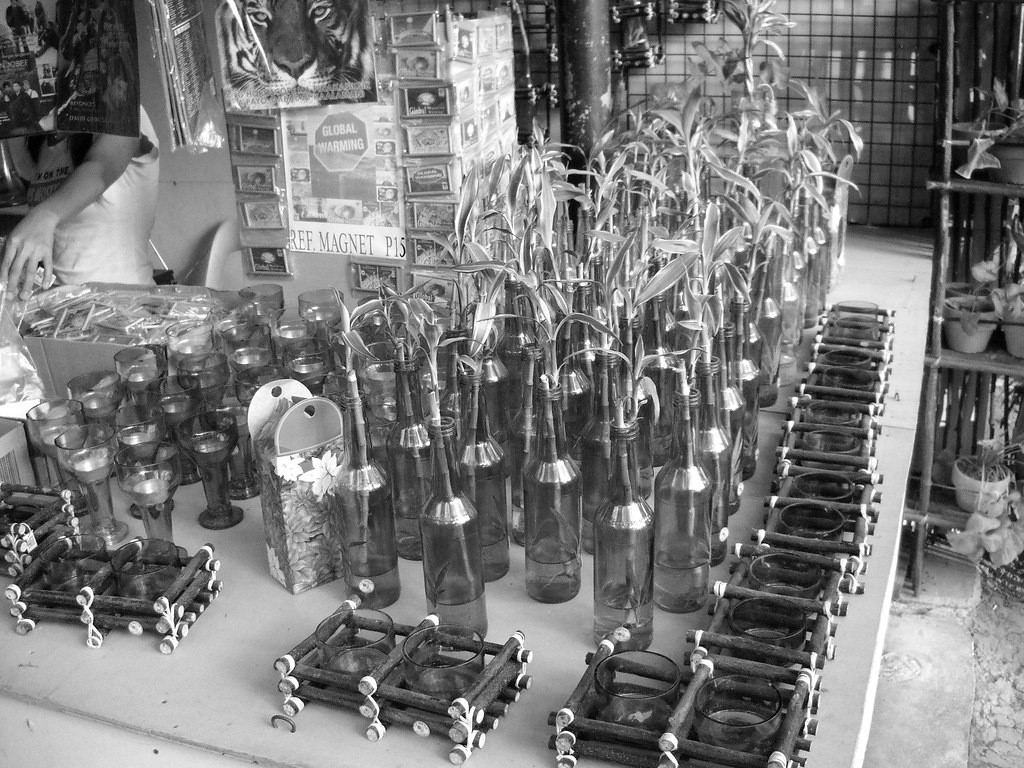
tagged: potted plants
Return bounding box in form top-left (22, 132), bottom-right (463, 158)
top-left (947, 76), bottom-right (1024, 518)
top-left (335, 0), bottom-right (861, 657)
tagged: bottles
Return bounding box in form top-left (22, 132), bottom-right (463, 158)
top-left (332, 121), bottom-right (835, 651)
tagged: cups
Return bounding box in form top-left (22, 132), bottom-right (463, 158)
top-left (791, 470), bottom-right (856, 504)
top-left (594, 650), bottom-right (682, 750)
top-left (357, 340), bottom-right (400, 422)
top-left (746, 552), bottom-right (824, 600)
top-left (834, 300), bottom-right (878, 336)
top-left (823, 348), bottom-right (871, 370)
top-left (777, 502), bottom-right (845, 541)
top-left (832, 315), bottom-right (879, 350)
top-left (694, 673), bottom-right (783, 756)
top-left (315, 607), bottom-right (396, 698)
top-left (802, 429), bottom-right (862, 456)
top-left (39, 534), bottom-right (119, 598)
top-left (296, 373), bottom-right (356, 466)
top-left (402, 624), bottom-right (485, 717)
top-left (728, 596), bottom-right (808, 668)
top-left (805, 400), bottom-right (860, 427)
top-left (821, 366), bottom-right (874, 404)
top-left (110, 538), bottom-right (181, 601)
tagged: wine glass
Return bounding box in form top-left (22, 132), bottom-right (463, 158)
top-left (25, 283), bottom-right (403, 562)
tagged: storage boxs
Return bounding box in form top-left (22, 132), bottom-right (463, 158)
top-left (21, 275), bottom-right (250, 401)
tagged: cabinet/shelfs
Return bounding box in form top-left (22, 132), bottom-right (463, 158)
top-left (904, 0), bottom-right (1024, 600)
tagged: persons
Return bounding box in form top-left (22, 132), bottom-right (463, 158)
top-left (0, 0), bottom-right (160, 302)
top-left (0, 0), bottom-right (59, 128)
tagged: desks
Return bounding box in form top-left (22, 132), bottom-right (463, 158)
top-left (0, 223), bottom-right (936, 766)
